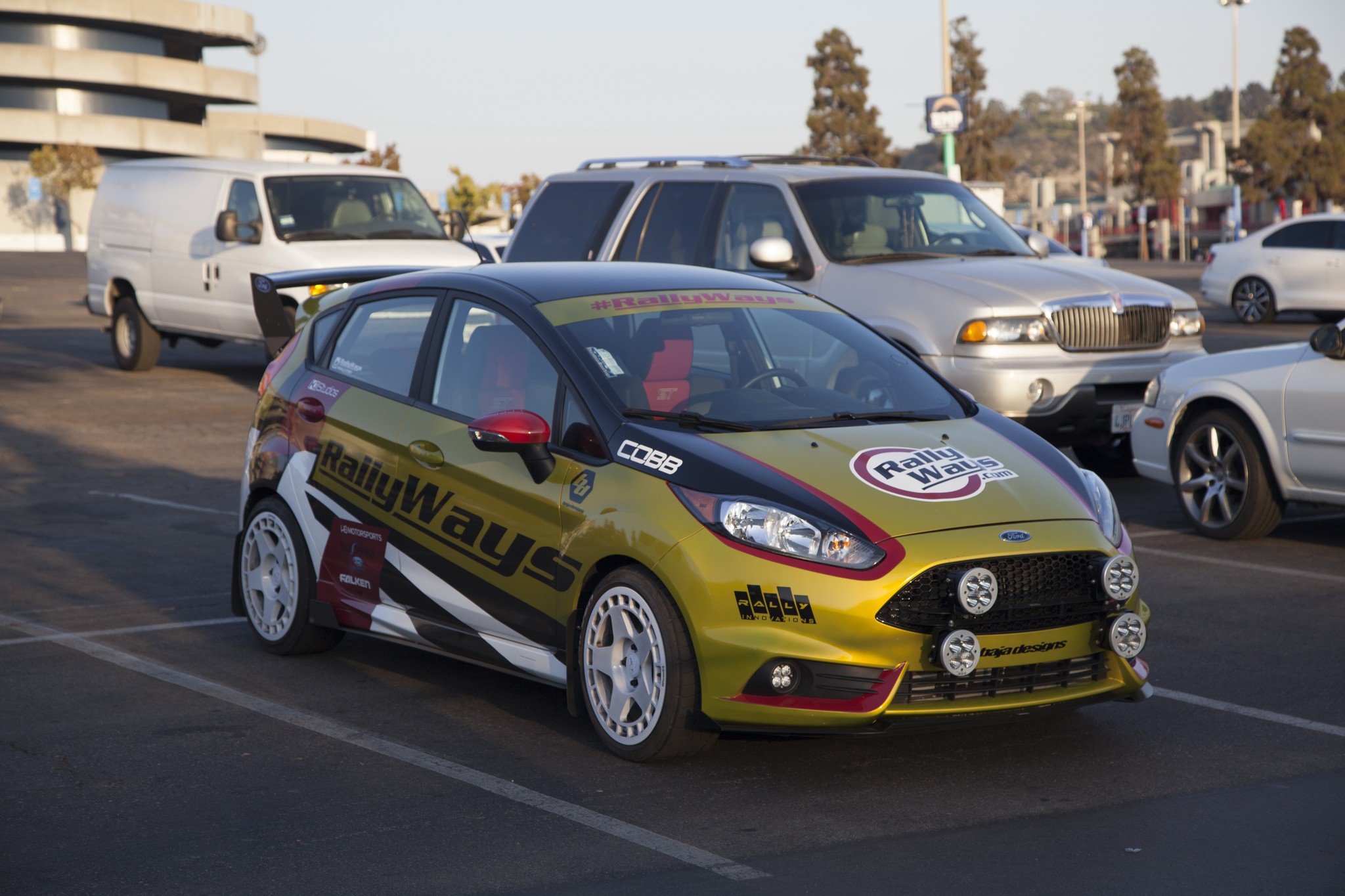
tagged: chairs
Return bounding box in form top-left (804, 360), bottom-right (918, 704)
top-left (733, 218), bottom-right (784, 272)
top-left (333, 198), bottom-right (371, 227)
top-left (446, 324), bottom-right (537, 418)
top-left (842, 223), bottom-right (894, 257)
top-left (620, 319), bottom-right (693, 421)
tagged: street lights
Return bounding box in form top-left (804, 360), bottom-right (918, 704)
top-left (1218, 0), bottom-right (1249, 230)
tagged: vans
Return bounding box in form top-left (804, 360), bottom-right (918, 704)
top-left (84, 156), bottom-right (486, 372)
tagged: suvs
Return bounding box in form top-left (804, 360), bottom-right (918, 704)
top-left (509, 152), bottom-right (1209, 479)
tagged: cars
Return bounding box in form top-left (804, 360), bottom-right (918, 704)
top-left (228, 262), bottom-right (1161, 766)
top-left (461, 234), bottom-right (512, 263)
top-left (1199, 210), bottom-right (1345, 324)
top-left (1128, 315), bottom-right (1345, 540)
